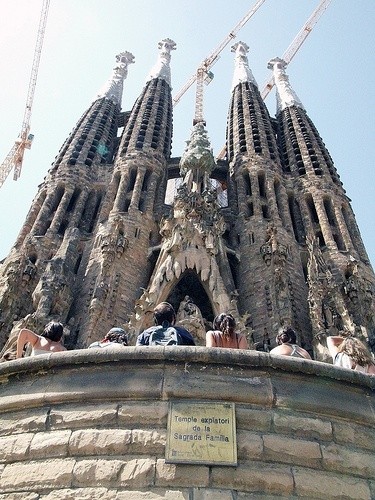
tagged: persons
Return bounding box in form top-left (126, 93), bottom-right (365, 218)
top-left (327, 336), bottom-right (375, 374)
top-left (136, 302), bottom-right (195, 346)
top-left (88, 328), bottom-right (128, 348)
top-left (206, 313), bottom-right (246, 349)
top-left (269, 326), bottom-right (312, 360)
top-left (17, 320), bottom-right (67, 358)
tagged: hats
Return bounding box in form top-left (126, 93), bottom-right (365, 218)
top-left (109, 328), bottom-right (127, 341)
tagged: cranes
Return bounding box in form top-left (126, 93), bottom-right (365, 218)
top-left (0, 0), bottom-right (51, 188)
top-left (218, 0), bottom-right (331, 161)
top-left (172, 1), bottom-right (266, 120)
